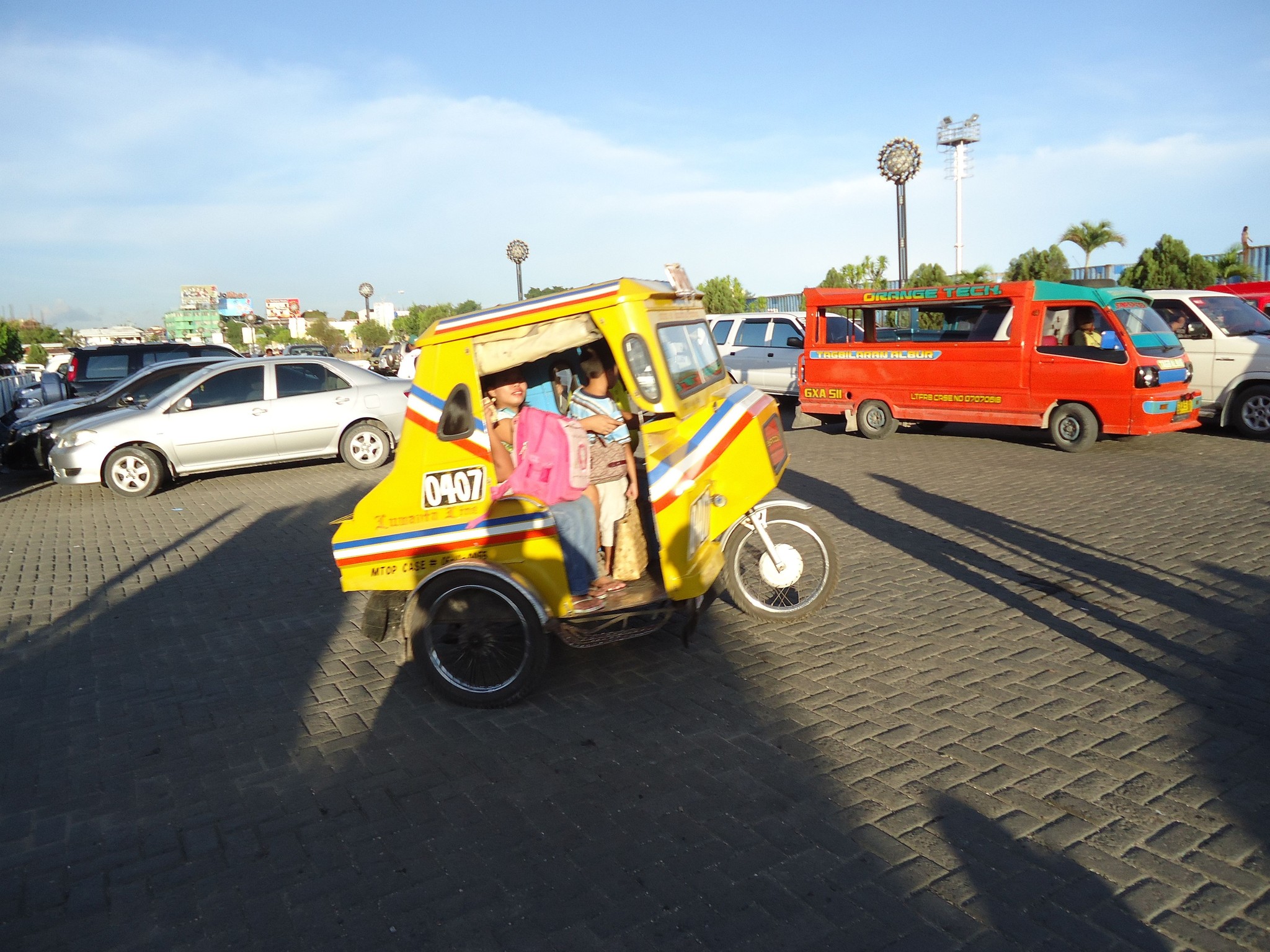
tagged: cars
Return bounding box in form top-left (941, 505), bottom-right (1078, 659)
top-left (49, 355), bottom-right (419, 499)
top-left (3, 357), bottom-right (324, 476)
top-left (347, 360), bottom-right (372, 371)
top-left (849, 318), bottom-right (891, 341)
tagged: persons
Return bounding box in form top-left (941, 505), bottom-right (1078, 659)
top-left (1072, 308), bottom-right (1102, 349)
top-left (398, 335), bottom-right (421, 380)
top-left (484, 342), bottom-right (656, 614)
top-left (262, 349), bottom-right (275, 357)
top-left (1241, 226), bottom-right (1253, 247)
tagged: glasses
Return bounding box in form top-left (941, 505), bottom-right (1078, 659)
top-left (411, 343), bottom-right (415, 347)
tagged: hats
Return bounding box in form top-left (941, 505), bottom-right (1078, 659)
top-left (408, 335), bottom-right (418, 345)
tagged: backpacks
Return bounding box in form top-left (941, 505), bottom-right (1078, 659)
top-left (463, 406), bottom-right (592, 531)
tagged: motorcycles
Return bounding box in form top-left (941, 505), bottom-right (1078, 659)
top-left (331, 265), bottom-right (840, 711)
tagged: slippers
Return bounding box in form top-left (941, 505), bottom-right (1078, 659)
top-left (572, 596), bottom-right (604, 613)
top-left (588, 586), bottom-right (608, 600)
top-left (596, 580), bottom-right (626, 593)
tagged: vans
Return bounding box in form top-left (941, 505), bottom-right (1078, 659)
top-left (797, 278), bottom-right (1203, 452)
top-left (991, 290), bottom-right (1270, 439)
top-left (1192, 281), bottom-right (1270, 329)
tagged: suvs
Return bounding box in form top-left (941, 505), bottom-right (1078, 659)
top-left (283, 345), bottom-right (328, 356)
top-left (40, 342), bottom-right (246, 405)
top-left (705, 311), bottom-right (863, 396)
top-left (367, 346), bottom-right (394, 371)
top-left (12, 359), bottom-right (70, 408)
top-left (45, 353), bottom-right (71, 378)
top-left (377, 341), bottom-right (412, 376)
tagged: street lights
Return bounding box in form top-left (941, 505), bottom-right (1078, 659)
top-left (182, 301), bottom-right (299, 354)
top-left (877, 138), bottom-right (922, 328)
top-left (358, 282), bottom-right (374, 320)
top-left (506, 239), bottom-right (529, 302)
top-left (944, 114), bottom-right (979, 275)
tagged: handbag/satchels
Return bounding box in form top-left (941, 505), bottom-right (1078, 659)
top-left (587, 430), bottom-right (627, 486)
top-left (611, 495), bottom-right (649, 581)
